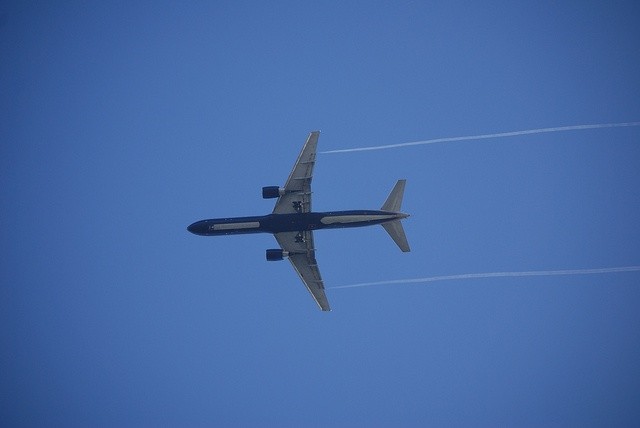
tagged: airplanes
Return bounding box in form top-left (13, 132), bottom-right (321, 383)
top-left (187, 130), bottom-right (411, 311)
